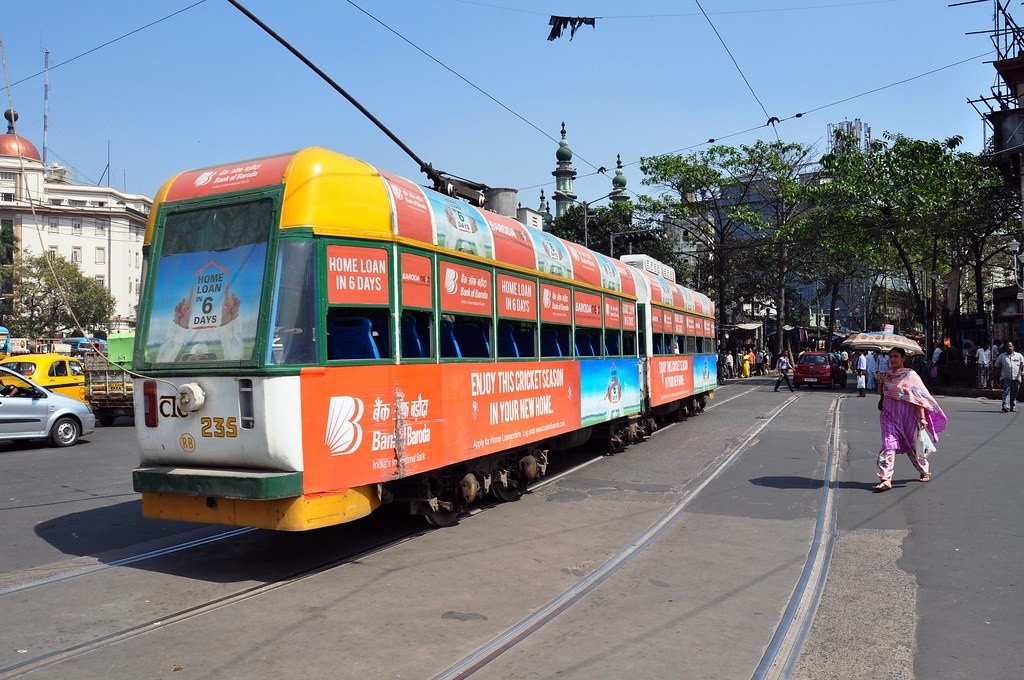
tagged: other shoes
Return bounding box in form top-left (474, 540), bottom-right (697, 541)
top-left (720, 383), bottom-right (725, 385)
top-left (791, 388), bottom-right (798, 393)
top-left (1002, 406), bottom-right (1010, 413)
top-left (774, 388), bottom-right (780, 392)
top-left (1012, 408), bottom-right (1017, 412)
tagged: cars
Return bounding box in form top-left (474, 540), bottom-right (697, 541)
top-left (793, 351), bottom-right (847, 390)
top-left (0, 351), bottom-right (89, 405)
top-left (0, 365), bottom-right (96, 448)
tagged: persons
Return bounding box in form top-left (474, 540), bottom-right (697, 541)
top-left (1, 364), bottom-right (33, 376)
top-left (898, 330), bottom-right (1024, 390)
top-left (716, 323), bottom-right (890, 397)
top-left (874, 347), bottom-right (947, 490)
top-left (154, 281), bottom-right (244, 363)
top-left (0, 379), bottom-right (18, 397)
top-left (668, 336), bottom-right (679, 354)
top-left (994, 340), bottom-right (1024, 412)
top-left (52, 361), bottom-right (77, 376)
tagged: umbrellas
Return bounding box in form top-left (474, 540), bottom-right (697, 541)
top-left (841, 331), bottom-right (923, 354)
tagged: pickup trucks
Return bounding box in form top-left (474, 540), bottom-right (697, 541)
top-left (19, 337), bottom-right (107, 376)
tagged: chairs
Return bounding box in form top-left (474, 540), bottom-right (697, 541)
top-left (331, 313), bottom-right (668, 359)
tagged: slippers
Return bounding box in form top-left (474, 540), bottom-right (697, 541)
top-left (918, 471), bottom-right (931, 482)
top-left (873, 482), bottom-right (893, 491)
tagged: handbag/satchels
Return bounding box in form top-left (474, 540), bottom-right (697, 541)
top-left (917, 428), bottom-right (935, 459)
top-left (878, 371), bottom-right (887, 411)
top-left (857, 375), bottom-right (866, 389)
top-left (930, 366), bottom-right (936, 377)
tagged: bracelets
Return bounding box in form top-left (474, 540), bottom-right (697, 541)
top-left (920, 418), bottom-right (925, 420)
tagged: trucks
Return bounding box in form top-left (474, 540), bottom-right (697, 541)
top-left (83, 372), bottom-right (134, 426)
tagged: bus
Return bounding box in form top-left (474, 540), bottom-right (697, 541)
top-left (129, 146), bottom-right (717, 536)
